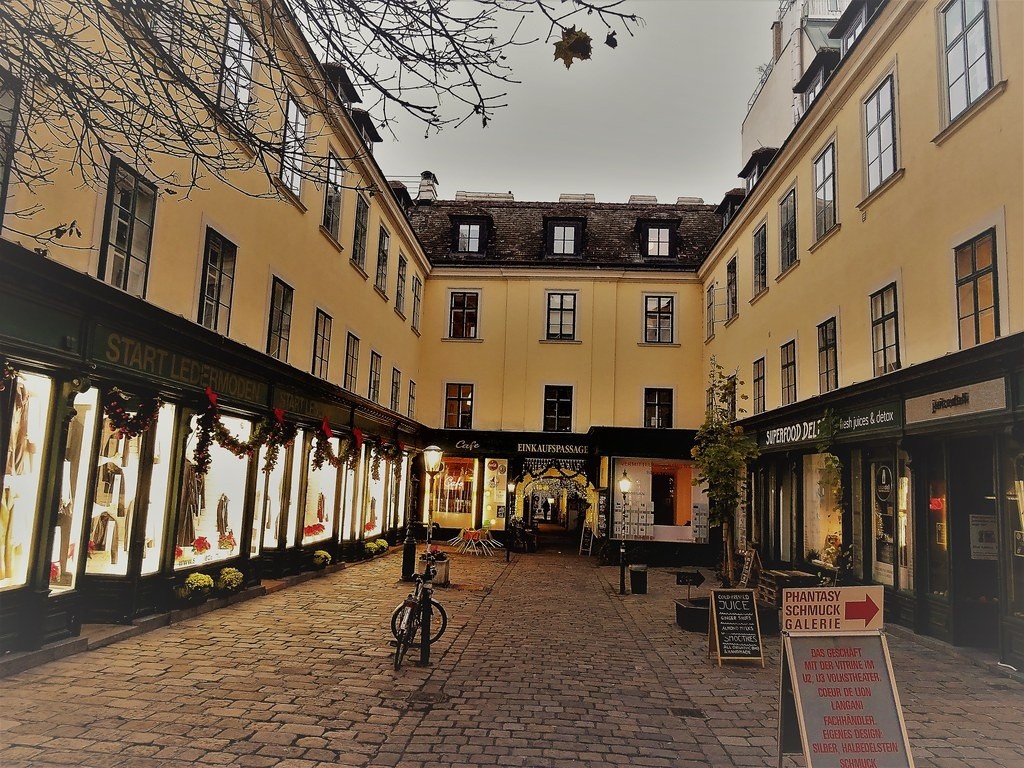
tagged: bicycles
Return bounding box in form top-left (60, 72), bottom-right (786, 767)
top-left (390, 559), bottom-right (447, 673)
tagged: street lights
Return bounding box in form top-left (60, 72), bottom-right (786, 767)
top-left (507, 481), bottom-right (515, 561)
top-left (420, 445), bottom-right (445, 574)
top-left (618, 469), bottom-right (633, 594)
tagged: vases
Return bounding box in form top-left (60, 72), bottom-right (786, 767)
top-left (194, 587), bottom-right (210, 596)
top-left (418, 559), bottom-right (450, 585)
top-left (378, 546), bottom-right (385, 554)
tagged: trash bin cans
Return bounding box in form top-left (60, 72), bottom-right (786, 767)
top-left (628, 564), bottom-right (648, 594)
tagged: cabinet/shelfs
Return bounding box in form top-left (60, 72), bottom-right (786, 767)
top-left (757, 569), bottom-right (816, 607)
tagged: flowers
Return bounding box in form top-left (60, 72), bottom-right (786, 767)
top-left (365, 542), bottom-right (378, 554)
top-left (313, 550), bottom-right (332, 565)
top-left (186, 572), bottom-right (214, 590)
top-left (365, 522), bottom-right (375, 530)
top-left (218, 567), bottom-right (243, 589)
top-left (375, 539), bottom-right (388, 549)
top-left (191, 536), bottom-right (210, 551)
top-left (174, 546), bottom-right (182, 557)
top-left (305, 522), bottom-right (325, 535)
top-left (418, 546), bottom-right (447, 561)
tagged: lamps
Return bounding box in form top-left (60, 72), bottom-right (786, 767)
top-left (422, 444), bottom-right (445, 473)
top-left (507, 479), bottom-right (516, 493)
top-left (619, 470), bottom-right (632, 494)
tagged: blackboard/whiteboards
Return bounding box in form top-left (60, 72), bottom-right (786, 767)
top-left (580, 526), bottom-right (594, 551)
top-left (710, 588), bottom-right (763, 660)
top-left (740, 549), bottom-right (756, 584)
